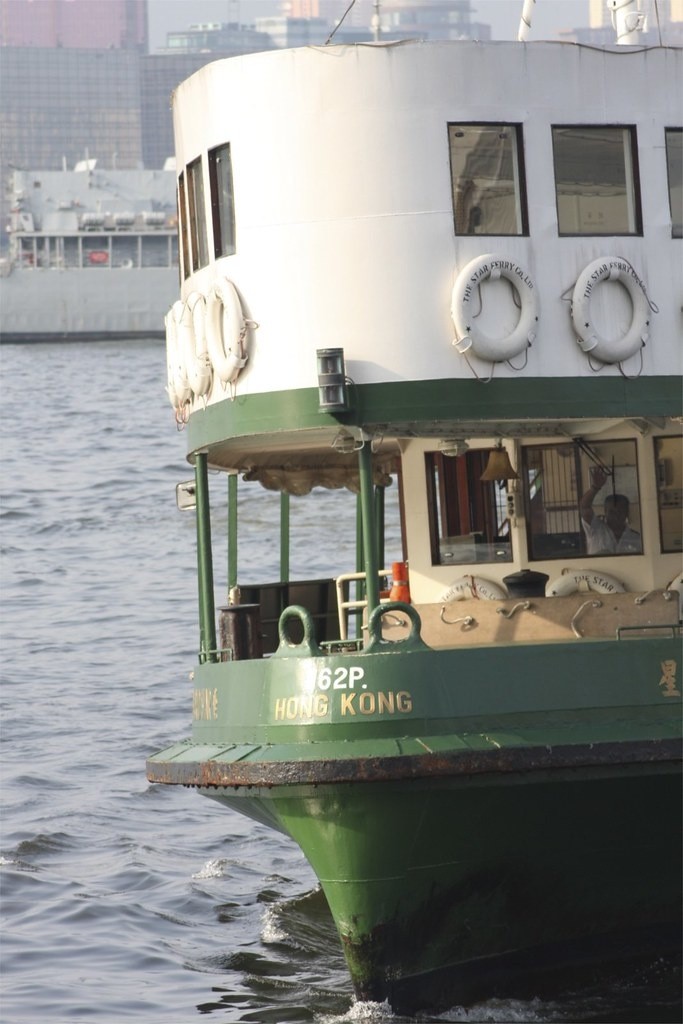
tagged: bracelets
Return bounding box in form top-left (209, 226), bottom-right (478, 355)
top-left (592, 484), bottom-right (601, 490)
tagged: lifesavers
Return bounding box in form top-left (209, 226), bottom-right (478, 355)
top-left (450, 253), bottom-right (540, 363)
top-left (547, 567), bottom-right (627, 595)
top-left (436, 575), bottom-right (508, 603)
top-left (205, 278), bottom-right (245, 382)
top-left (164, 292), bottom-right (210, 406)
top-left (571, 256), bottom-right (653, 364)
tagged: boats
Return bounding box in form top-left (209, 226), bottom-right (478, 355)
top-left (141, 0), bottom-right (683, 1006)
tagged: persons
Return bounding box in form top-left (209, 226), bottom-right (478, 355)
top-left (581, 465), bottom-right (644, 555)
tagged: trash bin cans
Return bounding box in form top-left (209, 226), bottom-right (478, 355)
top-left (216, 604), bottom-right (264, 663)
top-left (502, 568), bottom-right (550, 599)
